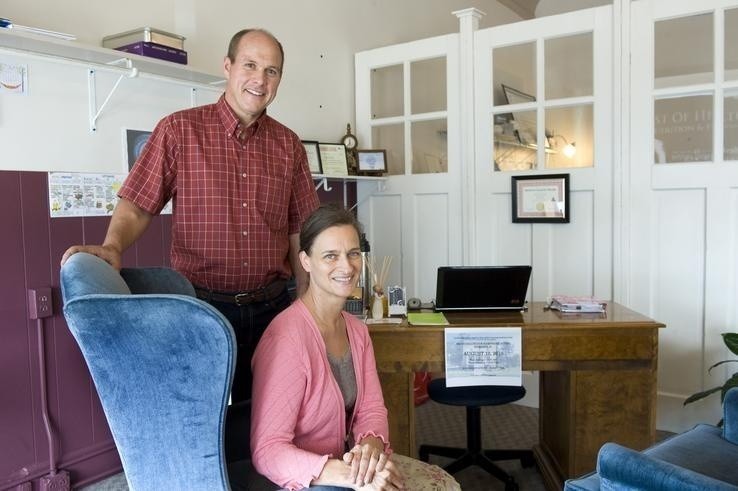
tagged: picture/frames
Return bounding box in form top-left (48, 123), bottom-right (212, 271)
top-left (299, 138), bottom-right (323, 175)
top-left (317, 141), bottom-right (350, 176)
top-left (355, 148), bottom-right (388, 174)
top-left (498, 81), bottom-right (551, 148)
top-left (510, 173), bottom-right (570, 224)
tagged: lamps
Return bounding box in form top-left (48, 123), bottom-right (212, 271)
top-left (546, 133), bottom-right (578, 158)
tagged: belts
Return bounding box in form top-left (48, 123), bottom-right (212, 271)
top-left (194, 275), bottom-right (289, 309)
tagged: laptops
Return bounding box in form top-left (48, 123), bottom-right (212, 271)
top-left (433, 264), bottom-right (532, 309)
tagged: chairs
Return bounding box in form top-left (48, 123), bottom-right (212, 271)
top-left (57, 248), bottom-right (281, 491)
top-left (558, 381), bottom-right (736, 491)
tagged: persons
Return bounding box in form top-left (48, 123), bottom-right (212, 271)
top-left (60, 26), bottom-right (323, 456)
top-left (248, 202), bottom-right (464, 491)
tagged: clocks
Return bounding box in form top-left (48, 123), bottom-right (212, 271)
top-left (341, 123), bottom-right (358, 150)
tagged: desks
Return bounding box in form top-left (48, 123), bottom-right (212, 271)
top-left (364, 297), bottom-right (670, 491)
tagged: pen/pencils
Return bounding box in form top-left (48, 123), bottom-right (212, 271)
top-left (363, 255), bottom-right (394, 289)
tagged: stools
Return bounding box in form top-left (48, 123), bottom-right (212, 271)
top-left (417, 376), bottom-right (536, 491)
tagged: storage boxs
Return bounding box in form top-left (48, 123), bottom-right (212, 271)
top-left (102, 25), bottom-right (186, 51)
top-left (112, 40), bottom-right (188, 64)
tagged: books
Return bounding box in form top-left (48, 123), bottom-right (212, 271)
top-left (405, 309), bottom-right (450, 327)
top-left (546, 294), bottom-right (608, 313)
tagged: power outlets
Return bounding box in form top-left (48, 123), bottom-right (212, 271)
top-left (26, 285), bottom-right (56, 321)
top-left (32, 469), bottom-right (72, 491)
